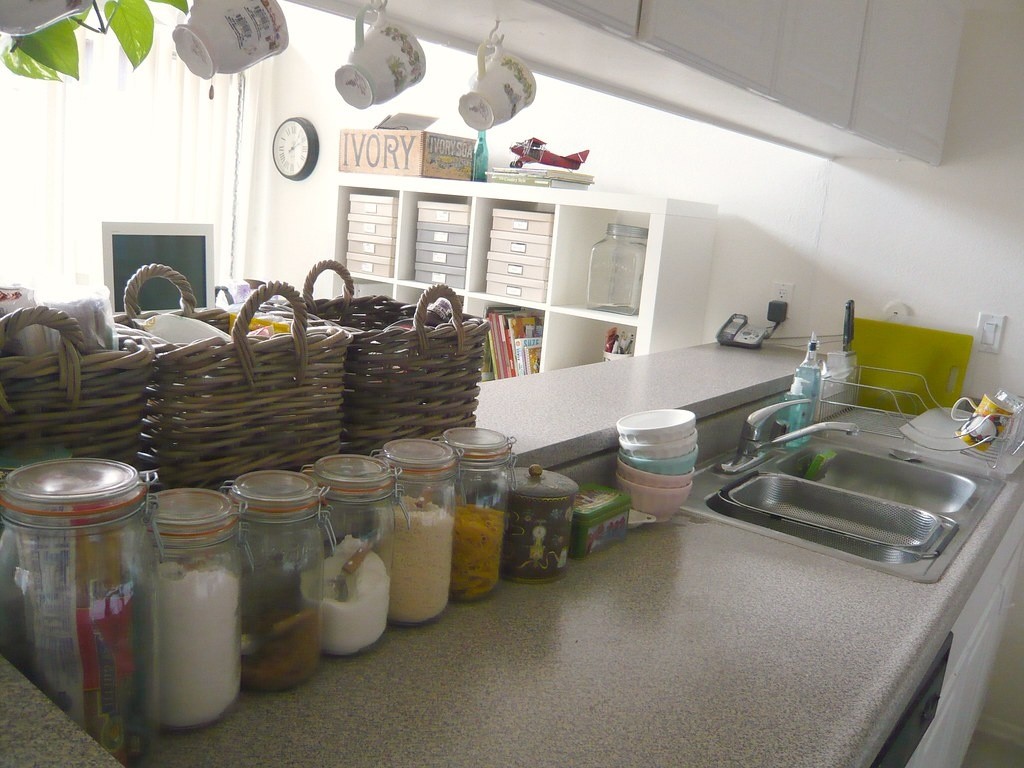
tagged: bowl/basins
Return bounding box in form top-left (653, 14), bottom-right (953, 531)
top-left (615, 409), bottom-right (698, 523)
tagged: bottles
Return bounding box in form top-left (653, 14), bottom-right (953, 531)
top-left (588, 223), bottom-right (648, 316)
top-left (471, 130), bottom-right (488, 182)
top-left (368, 438), bottom-right (465, 626)
top-left (300, 453), bottom-right (410, 656)
top-left (0, 457), bottom-right (160, 768)
top-left (381, 297), bottom-right (453, 331)
top-left (430, 426), bottom-right (518, 602)
top-left (150, 488), bottom-right (241, 731)
top-left (220, 469), bottom-right (338, 692)
top-left (795, 330), bottom-right (821, 442)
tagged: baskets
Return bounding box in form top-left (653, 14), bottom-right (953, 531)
top-left (114, 264), bottom-right (353, 493)
top-left (0, 305), bottom-right (152, 464)
top-left (284, 259), bottom-right (492, 457)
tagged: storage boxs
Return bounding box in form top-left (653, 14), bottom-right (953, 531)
top-left (338, 128), bottom-right (476, 181)
top-left (415, 242), bottom-right (467, 268)
top-left (348, 232), bottom-right (396, 257)
top-left (346, 214), bottom-right (397, 238)
top-left (346, 252), bottom-right (394, 278)
top-left (350, 193), bottom-right (399, 217)
top-left (490, 230), bottom-right (553, 259)
top-left (484, 272), bottom-right (548, 302)
top-left (486, 251), bottom-right (549, 283)
top-left (415, 222), bottom-right (469, 246)
top-left (491, 208), bottom-right (554, 236)
top-left (414, 263), bottom-right (467, 290)
top-left (416, 201), bottom-right (472, 227)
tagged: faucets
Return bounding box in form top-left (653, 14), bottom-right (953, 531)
top-left (713, 398), bottom-right (859, 475)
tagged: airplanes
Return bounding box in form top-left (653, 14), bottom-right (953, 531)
top-left (509, 136), bottom-right (590, 170)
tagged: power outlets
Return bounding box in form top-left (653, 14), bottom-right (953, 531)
top-left (772, 282), bottom-right (794, 319)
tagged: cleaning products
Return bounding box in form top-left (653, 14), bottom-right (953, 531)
top-left (780, 330), bottom-right (821, 449)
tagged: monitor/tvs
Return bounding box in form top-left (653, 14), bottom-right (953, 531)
top-left (101, 221), bottom-right (216, 321)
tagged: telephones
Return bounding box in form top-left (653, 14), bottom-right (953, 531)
top-left (717, 313), bottom-right (769, 348)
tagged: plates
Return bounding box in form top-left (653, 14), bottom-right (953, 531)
top-left (627, 508), bottom-right (657, 529)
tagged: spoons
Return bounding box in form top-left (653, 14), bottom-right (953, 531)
top-left (325, 537), bottom-right (376, 602)
top-left (888, 450), bottom-right (917, 461)
top-left (241, 608), bottom-right (316, 655)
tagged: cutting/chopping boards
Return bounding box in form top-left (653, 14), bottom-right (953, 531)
top-left (847, 317), bottom-right (974, 416)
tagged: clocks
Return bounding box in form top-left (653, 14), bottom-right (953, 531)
top-left (272, 117), bottom-right (319, 181)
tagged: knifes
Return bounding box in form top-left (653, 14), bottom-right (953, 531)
top-left (843, 300), bottom-right (854, 351)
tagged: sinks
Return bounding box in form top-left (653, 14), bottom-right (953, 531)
top-left (701, 469), bottom-right (945, 569)
top-left (776, 443), bottom-right (977, 514)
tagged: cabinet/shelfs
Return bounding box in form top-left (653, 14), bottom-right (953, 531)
top-left (871, 502), bottom-right (1024, 768)
top-left (328, 172), bottom-right (720, 383)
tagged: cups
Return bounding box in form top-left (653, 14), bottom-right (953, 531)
top-left (172, 0), bottom-right (289, 80)
top-left (994, 388), bottom-right (1024, 415)
top-left (603, 350), bottom-right (633, 363)
top-left (951, 393), bottom-right (1013, 452)
top-left (458, 34), bottom-right (536, 130)
top-left (335, 0), bottom-right (426, 109)
top-left (0, 0), bottom-right (93, 34)
top-left (498, 464), bottom-right (578, 585)
